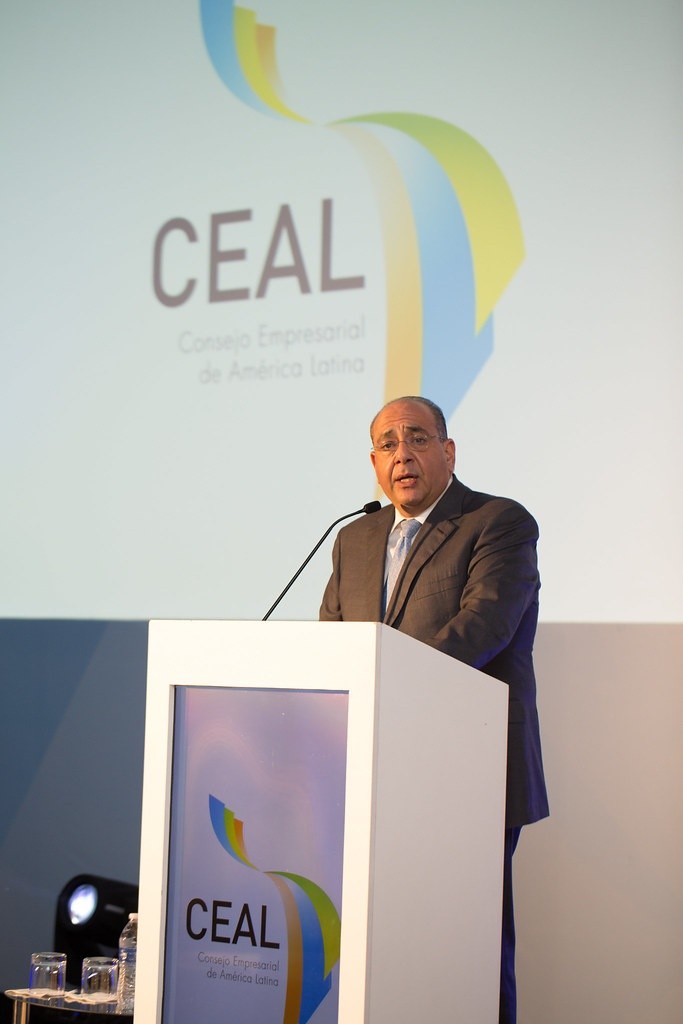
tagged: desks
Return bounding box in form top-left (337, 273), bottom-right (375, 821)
top-left (5, 989), bottom-right (134, 1024)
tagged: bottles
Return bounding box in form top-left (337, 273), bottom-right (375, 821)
top-left (116, 912), bottom-right (137, 1014)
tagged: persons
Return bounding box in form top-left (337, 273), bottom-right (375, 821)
top-left (319, 397), bottom-right (549, 1023)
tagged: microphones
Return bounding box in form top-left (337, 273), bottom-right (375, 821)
top-left (261, 500), bottom-right (381, 622)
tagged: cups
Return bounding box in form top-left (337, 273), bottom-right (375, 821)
top-left (80, 955), bottom-right (119, 1003)
top-left (28, 952), bottom-right (67, 999)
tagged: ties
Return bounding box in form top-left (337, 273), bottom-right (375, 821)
top-left (381, 518), bottom-right (423, 622)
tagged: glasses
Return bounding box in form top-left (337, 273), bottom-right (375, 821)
top-left (371, 428), bottom-right (448, 452)
